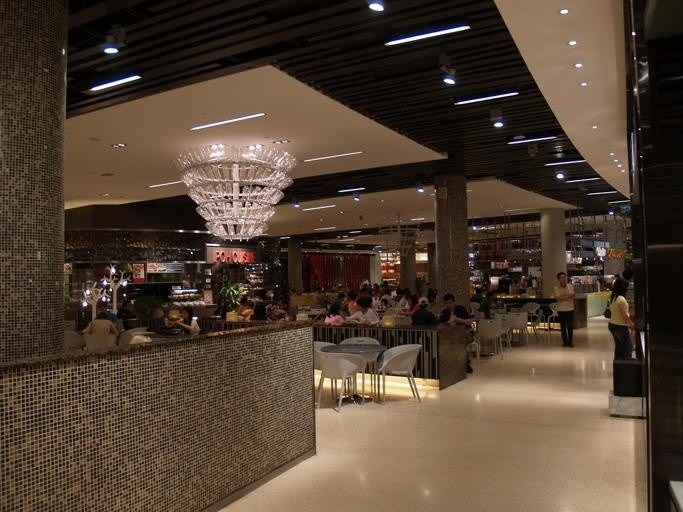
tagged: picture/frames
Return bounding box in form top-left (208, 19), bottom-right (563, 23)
top-left (131, 263), bottom-right (145, 284)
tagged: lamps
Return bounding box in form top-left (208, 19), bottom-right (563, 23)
top-left (527, 144), bottom-right (538, 158)
top-left (416, 182), bottom-right (425, 193)
top-left (488, 108), bottom-right (506, 128)
top-left (352, 191), bottom-right (360, 201)
top-left (102, 34), bottom-right (119, 53)
top-left (435, 52), bottom-right (456, 86)
top-left (171, 141), bottom-right (297, 243)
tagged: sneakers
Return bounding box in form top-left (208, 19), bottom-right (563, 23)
top-left (561, 343), bottom-right (575, 348)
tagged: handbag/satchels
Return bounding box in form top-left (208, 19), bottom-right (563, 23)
top-left (603, 308), bottom-right (611, 318)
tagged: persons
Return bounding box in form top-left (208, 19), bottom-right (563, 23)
top-left (620, 269), bottom-right (636, 351)
top-left (215, 280), bottom-right (299, 330)
top-left (95, 302), bottom-right (116, 321)
top-left (609, 274), bottom-right (619, 288)
top-left (478, 293), bottom-right (497, 319)
top-left (604, 279), bottom-right (635, 360)
top-left (177, 305), bottom-right (201, 333)
top-left (81, 313), bottom-right (118, 336)
top-left (313, 274), bottom-right (474, 373)
top-left (116, 299), bottom-right (138, 320)
top-left (470, 272), bottom-right (533, 303)
top-left (552, 271), bottom-right (575, 347)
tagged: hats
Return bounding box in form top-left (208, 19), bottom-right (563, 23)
top-left (418, 296), bottom-right (429, 305)
